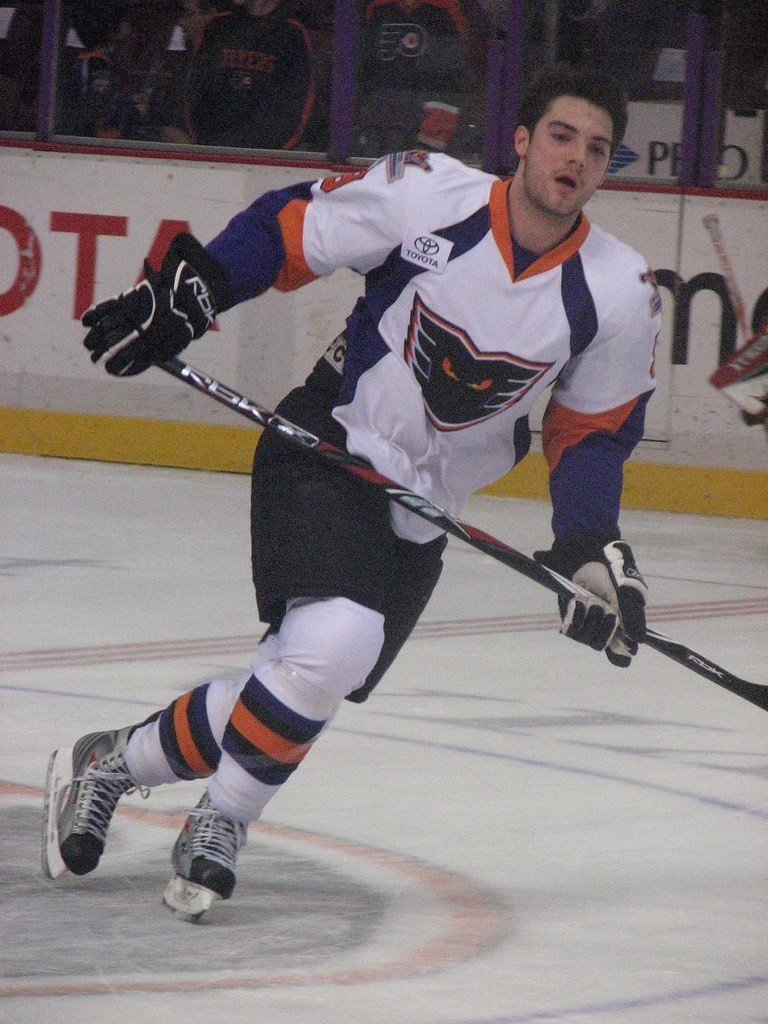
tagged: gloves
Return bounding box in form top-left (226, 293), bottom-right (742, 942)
top-left (533, 522), bottom-right (650, 668)
top-left (81, 232), bottom-right (236, 376)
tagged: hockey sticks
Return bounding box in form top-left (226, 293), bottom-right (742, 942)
top-left (703, 213), bottom-right (751, 346)
top-left (156, 356), bottom-right (768, 712)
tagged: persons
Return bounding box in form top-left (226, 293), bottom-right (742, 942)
top-left (0, 0), bottom-right (507, 163)
top-left (42, 64), bottom-right (664, 922)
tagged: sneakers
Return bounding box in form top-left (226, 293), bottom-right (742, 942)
top-left (161, 789), bottom-right (249, 921)
top-left (40, 725), bottom-right (151, 882)
